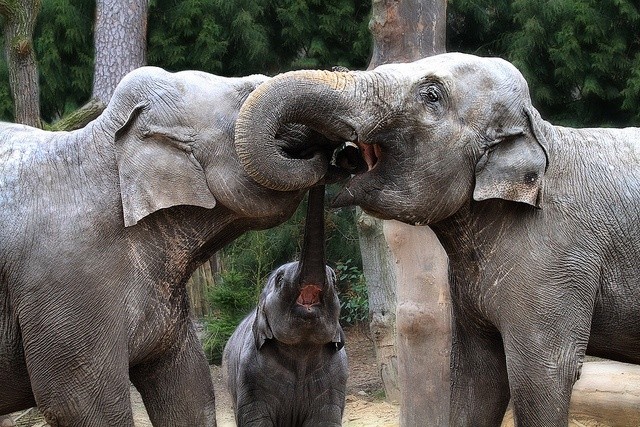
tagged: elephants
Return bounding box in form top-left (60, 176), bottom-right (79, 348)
top-left (0, 66), bottom-right (345, 426)
top-left (236, 53), bottom-right (640, 426)
top-left (222, 185), bottom-right (349, 426)
